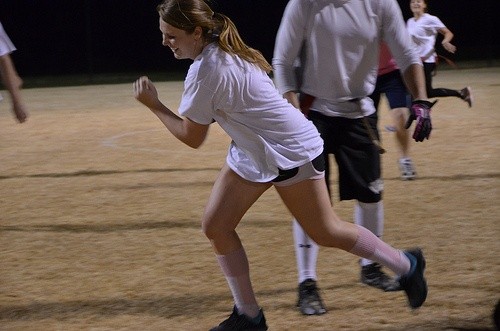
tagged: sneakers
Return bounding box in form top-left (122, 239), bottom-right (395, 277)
top-left (361, 262), bottom-right (402, 292)
top-left (395, 248), bottom-right (428, 309)
top-left (400, 157), bottom-right (417, 181)
top-left (461, 86), bottom-right (472, 107)
top-left (384, 125), bottom-right (397, 132)
top-left (298, 278), bottom-right (327, 316)
top-left (209, 304), bottom-right (268, 331)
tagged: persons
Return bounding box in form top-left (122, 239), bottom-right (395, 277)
top-left (274, 0), bottom-right (472, 315)
top-left (134, 0), bottom-right (428, 331)
top-left (0, 23), bottom-right (29, 124)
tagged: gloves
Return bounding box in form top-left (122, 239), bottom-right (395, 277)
top-left (404, 99), bottom-right (438, 142)
